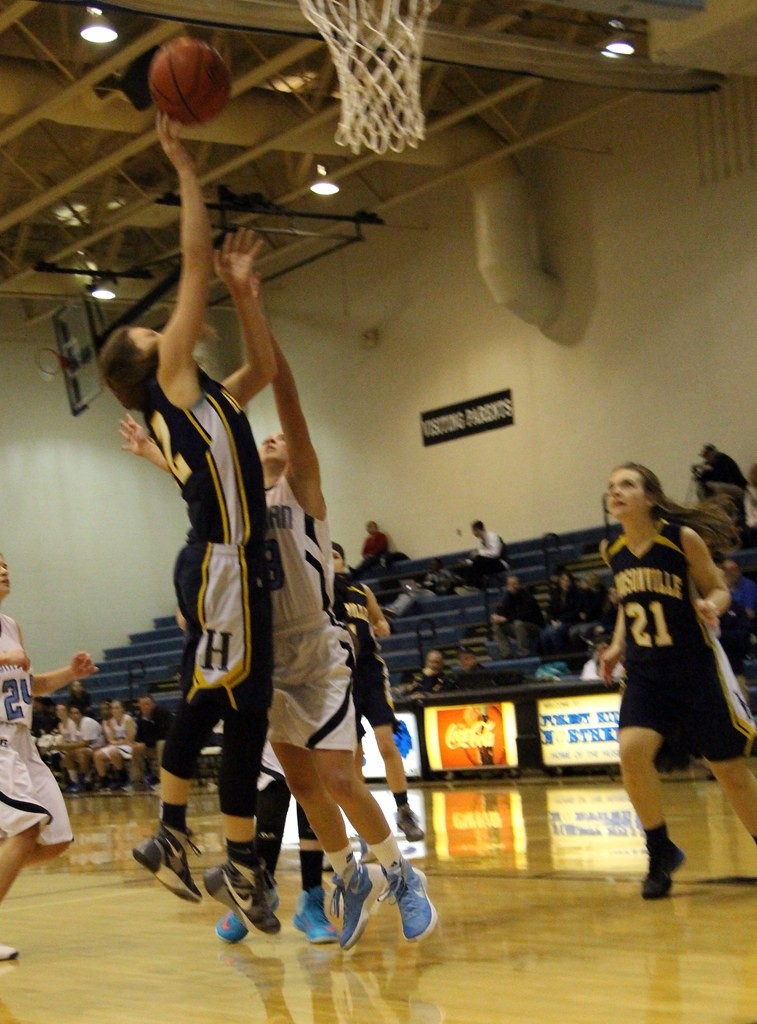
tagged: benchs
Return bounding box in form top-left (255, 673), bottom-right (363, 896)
top-left (30, 511), bottom-right (756, 787)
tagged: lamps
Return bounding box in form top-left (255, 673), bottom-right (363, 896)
top-left (81, 7), bottom-right (117, 43)
top-left (91, 279), bottom-right (116, 300)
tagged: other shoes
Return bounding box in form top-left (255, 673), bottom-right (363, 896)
top-left (0, 945), bottom-right (18, 960)
top-left (324, 853), bottom-right (353, 872)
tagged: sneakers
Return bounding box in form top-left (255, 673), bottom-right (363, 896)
top-left (396, 803), bottom-right (424, 842)
top-left (132, 821), bottom-right (203, 903)
top-left (642, 841), bottom-right (685, 899)
top-left (329, 862), bottom-right (386, 951)
top-left (203, 858), bottom-right (281, 937)
top-left (216, 887), bottom-right (279, 943)
top-left (292, 886), bottom-right (338, 944)
top-left (377, 854), bottom-right (437, 943)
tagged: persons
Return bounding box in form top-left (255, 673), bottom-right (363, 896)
top-left (331, 541), bottom-right (425, 842)
top-left (380, 557), bottom-right (453, 620)
top-left (117, 268), bottom-right (438, 950)
top-left (392, 647), bottom-right (520, 781)
top-left (349, 520), bottom-right (388, 580)
top-left (457, 521), bottom-right (509, 595)
top-left (31, 682), bottom-right (173, 792)
top-left (599, 463), bottom-right (757, 898)
top-left (218, 743), bottom-right (340, 942)
top-left (0, 554), bottom-right (98, 962)
top-left (104, 119), bottom-right (283, 936)
top-left (491, 574), bottom-right (626, 680)
top-left (690, 443), bottom-right (756, 706)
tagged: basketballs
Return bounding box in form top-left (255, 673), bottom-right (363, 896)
top-left (147, 37), bottom-right (231, 128)
